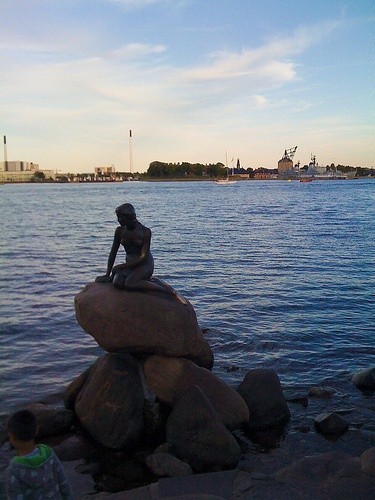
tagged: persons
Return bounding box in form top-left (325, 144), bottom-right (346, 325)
top-left (95, 202), bottom-right (188, 306)
top-left (4, 409), bottom-right (74, 500)
